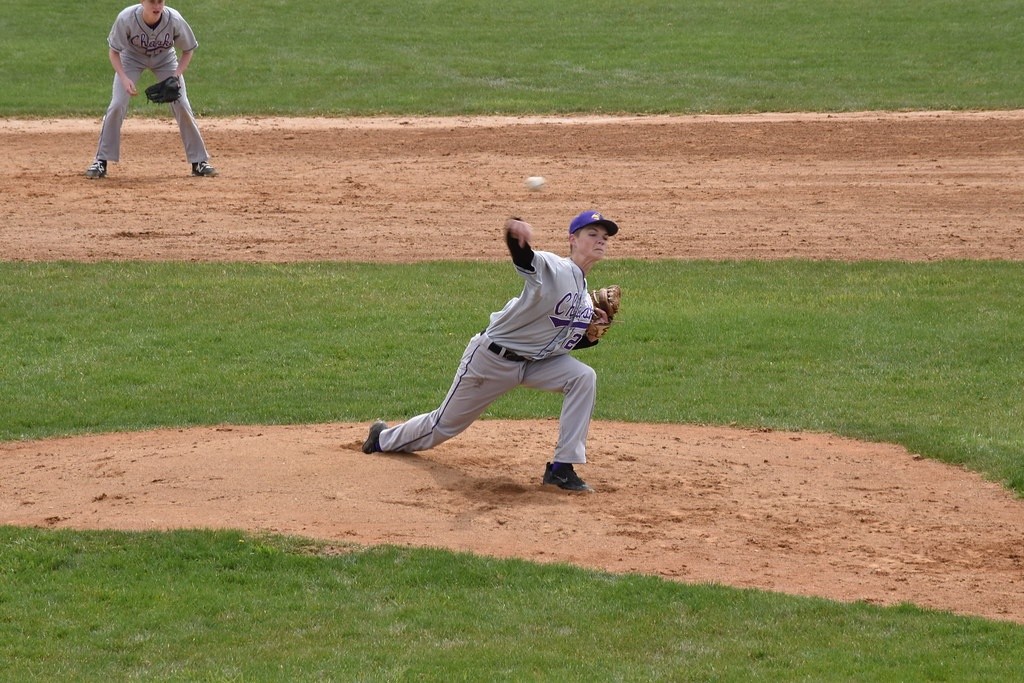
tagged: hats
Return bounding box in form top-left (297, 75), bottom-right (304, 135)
top-left (569, 211), bottom-right (619, 237)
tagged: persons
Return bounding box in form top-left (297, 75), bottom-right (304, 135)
top-left (86, 0), bottom-right (219, 178)
top-left (362, 211), bottom-right (622, 494)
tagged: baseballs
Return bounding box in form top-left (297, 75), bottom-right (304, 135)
top-left (526, 177), bottom-right (545, 191)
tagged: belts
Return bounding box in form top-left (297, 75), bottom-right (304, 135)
top-left (480, 329), bottom-right (526, 362)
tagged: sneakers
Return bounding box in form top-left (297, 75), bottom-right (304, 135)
top-left (362, 421), bottom-right (388, 454)
top-left (83, 159), bottom-right (107, 178)
top-left (543, 461), bottom-right (594, 493)
top-left (191, 160), bottom-right (219, 177)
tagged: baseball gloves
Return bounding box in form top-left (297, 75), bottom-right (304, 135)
top-left (145, 76), bottom-right (181, 104)
top-left (586, 285), bottom-right (622, 339)
